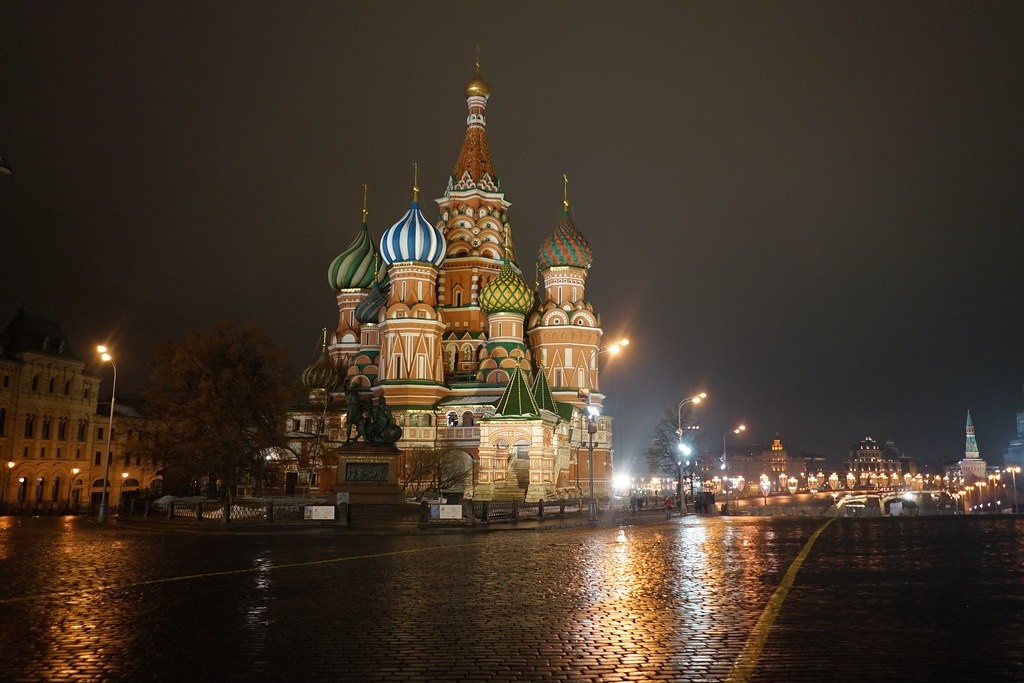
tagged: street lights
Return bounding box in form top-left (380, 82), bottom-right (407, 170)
top-left (989, 474), bottom-right (1001, 511)
top-left (97, 344), bottom-right (116, 523)
top-left (722, 425), bottom-right (747, 506)
top-left (1006, 467), bottom-right (1020, 498)
top-left (975, 482), bottom-right (986, 502)
top-left (678, 393), bottom-right (707, 514)
top-left (587, 337), bottom-right (630, 521)
top-left (965, 486), bottom-right (974, 503)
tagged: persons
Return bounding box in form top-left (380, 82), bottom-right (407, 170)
top-left (665, 495), bottom-right (673, 520)
top-left (637, 495), bottom-right (644, 513)
top-left (630, 493), bottom-right (637, 513)
top-left (344, 377), bottom-right (370, 443)
top-left (721, 504), bottom-right (725, 515)
top-left (694, 497), bottom-right (710, 514)
top-left (677, 499), bottom-right (682, 512)
top-left (347, 395), bottom-right (394, 444)
top-left (725, 504), bottom-right (728, 515)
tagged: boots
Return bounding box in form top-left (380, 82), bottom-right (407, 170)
top-left (665, 513), bottom-right (671, 519)
